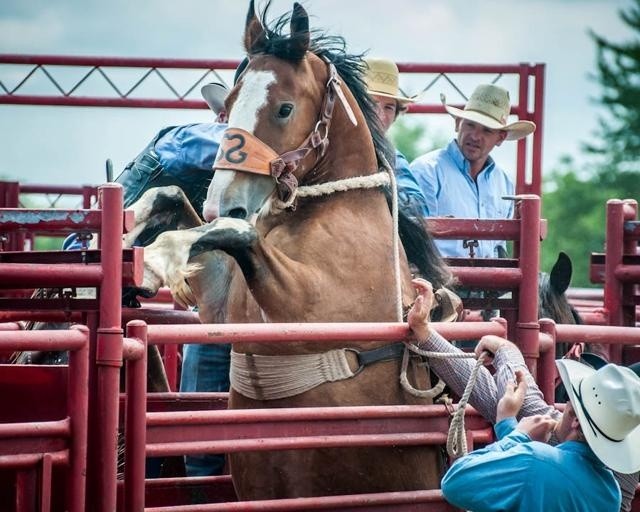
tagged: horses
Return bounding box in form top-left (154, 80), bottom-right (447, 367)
top-left (487, 246), bottom-right (593, 356)
top-left (109, 0), bottom-right (461, 509)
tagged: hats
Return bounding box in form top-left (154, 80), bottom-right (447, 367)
top-left (581, 353), bottom-right (640, 374)
top-left (440, 83), bottom-right (536, 140)
top-left (358, 56), bottom-right (423, 102)
top-left (201, 83), bottom-right (232, 114)
top-left (556, 358), bottom-right (640, 475)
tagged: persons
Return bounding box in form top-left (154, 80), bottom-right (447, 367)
top-left (407, 277), bottom-right (640, 511)
top-left (357, 55), bottom-right (425, 217)
top-left (410, 83), bottom-right (537, 347)
top-left (13, 80), bottom-right (234, 478)
top-left (441, 358), bottom-right (639, 511)
